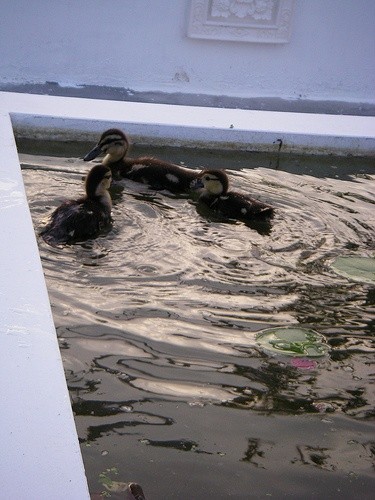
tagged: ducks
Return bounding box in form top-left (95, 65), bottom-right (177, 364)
top-left (82, 128), bottom-right (201, 195)
top-left (38, 165), bottom-right (112, 251)
top-left (190, 168), bottom-right (280, 236)
